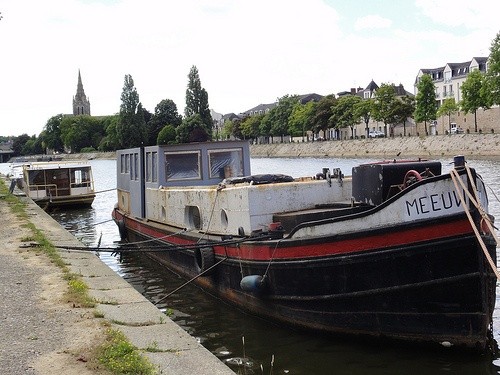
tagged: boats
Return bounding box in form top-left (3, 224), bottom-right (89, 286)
top-left (22, 161), bottom-right (96, 205)
top-left (111, 139), bottom-right (498, 359)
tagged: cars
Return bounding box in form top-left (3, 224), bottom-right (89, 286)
top-left (369, 131), bottom-right (385, 138)
